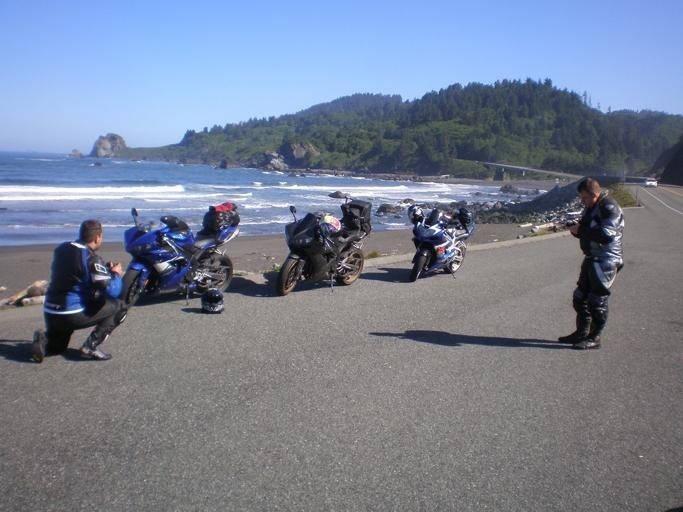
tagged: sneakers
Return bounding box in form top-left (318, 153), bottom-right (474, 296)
top-left (79, 345), bottom-right (111, 361)
top-left (558, 331), bottom-right (602, 351)
top-left (33, 330), bottom-right (46, 364)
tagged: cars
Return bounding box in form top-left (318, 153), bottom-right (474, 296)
top-left (644, 177), bottom-right (657, 187)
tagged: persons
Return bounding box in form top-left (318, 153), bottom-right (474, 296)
top-left (557, 176), bottom-right (627, 349)
top-left (29, 219), bottom-right (125, 363)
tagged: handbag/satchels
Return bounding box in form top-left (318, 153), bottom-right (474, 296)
top-left (202, 211), bottom-right (240, 232)
top-left (341, 200), bottom-right (371, 229)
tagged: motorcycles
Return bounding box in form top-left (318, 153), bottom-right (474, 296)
top-left (408, 202), bottom-right (475, 282)
top-left (277, 199), bottom-right (372, 296)
top-left (119, 203), bottom-right (239, 310)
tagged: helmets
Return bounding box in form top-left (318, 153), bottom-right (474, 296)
top-left (201, 289), bottom-right (225, 314)
top-left (408, 205), bottom-right (425, 223)
top-left (319, 215), bottom-right (342, 235)
